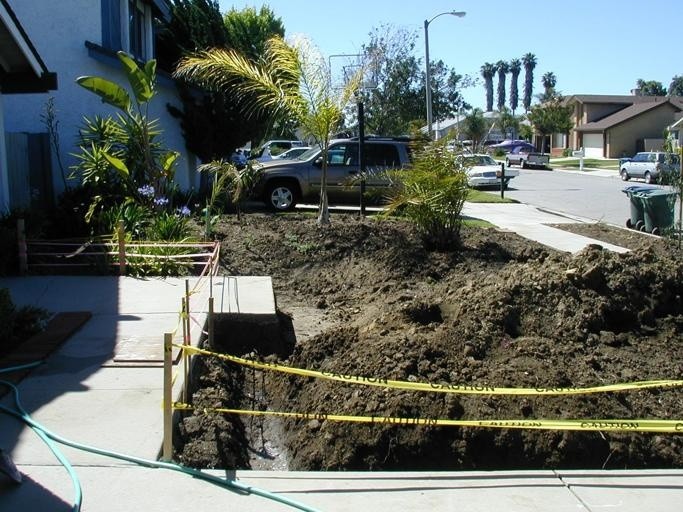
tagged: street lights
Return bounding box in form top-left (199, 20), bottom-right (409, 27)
top-left (423, 12), bottom-right (466, 139)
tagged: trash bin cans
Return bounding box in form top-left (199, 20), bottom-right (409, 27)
top-left (633, 189), bottom-right (678, 236)
top-left (622, 185), bottom-right (662, 230)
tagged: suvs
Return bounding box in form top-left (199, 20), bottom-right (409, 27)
top-left (619, 151), bottom-right (680, 184)
